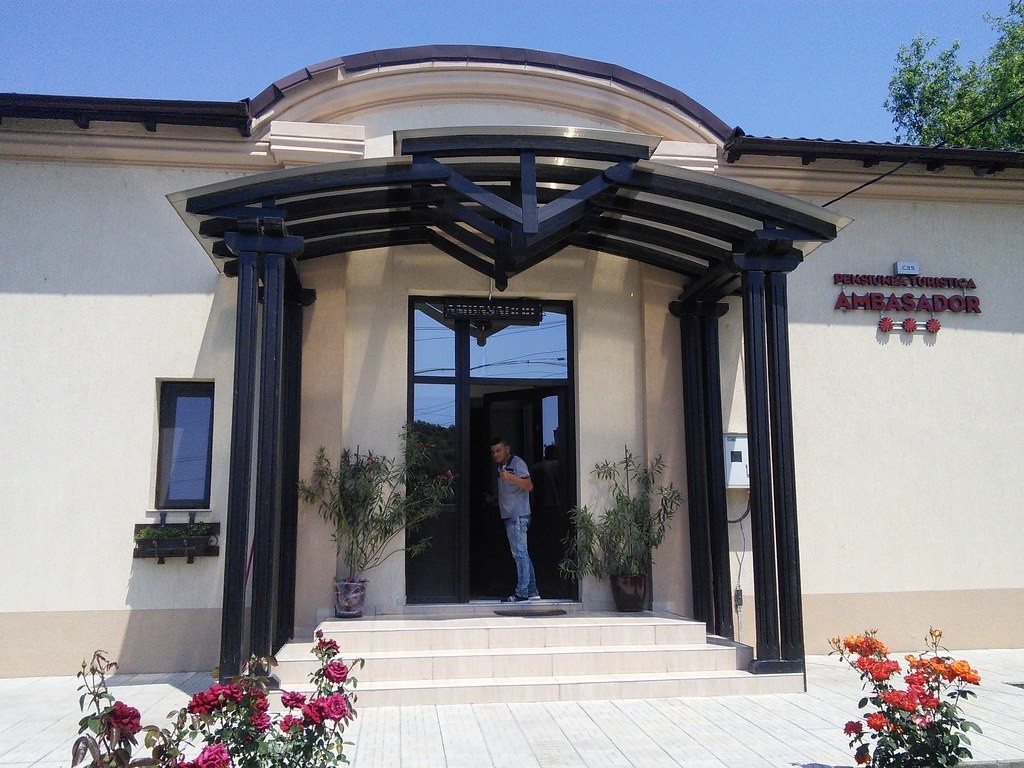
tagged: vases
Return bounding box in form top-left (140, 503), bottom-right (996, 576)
top-left (335, 582), bottom-right (365, 617)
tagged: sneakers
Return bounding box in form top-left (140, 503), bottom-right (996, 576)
top-left (501, 594), bottom-right (531, 604)
top-left (529, 593), bottom-right (541, 600)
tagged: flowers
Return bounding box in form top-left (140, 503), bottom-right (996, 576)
top-left (297, 423), bottom-right (454, 581)
top-left (827, 627), bottom-right (982, 768)
top-left (73, 629), bottom-right (365, 768)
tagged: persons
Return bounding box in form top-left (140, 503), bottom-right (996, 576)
top-left (491, 438), bottom-right (541, 604)
top-left (533, 445), bottom-right (559, 579)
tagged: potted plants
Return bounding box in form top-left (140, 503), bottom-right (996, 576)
top-left (133, 523), bottom-right (210, 550)
top-left (557, 442), bottom-right (686, 613)
